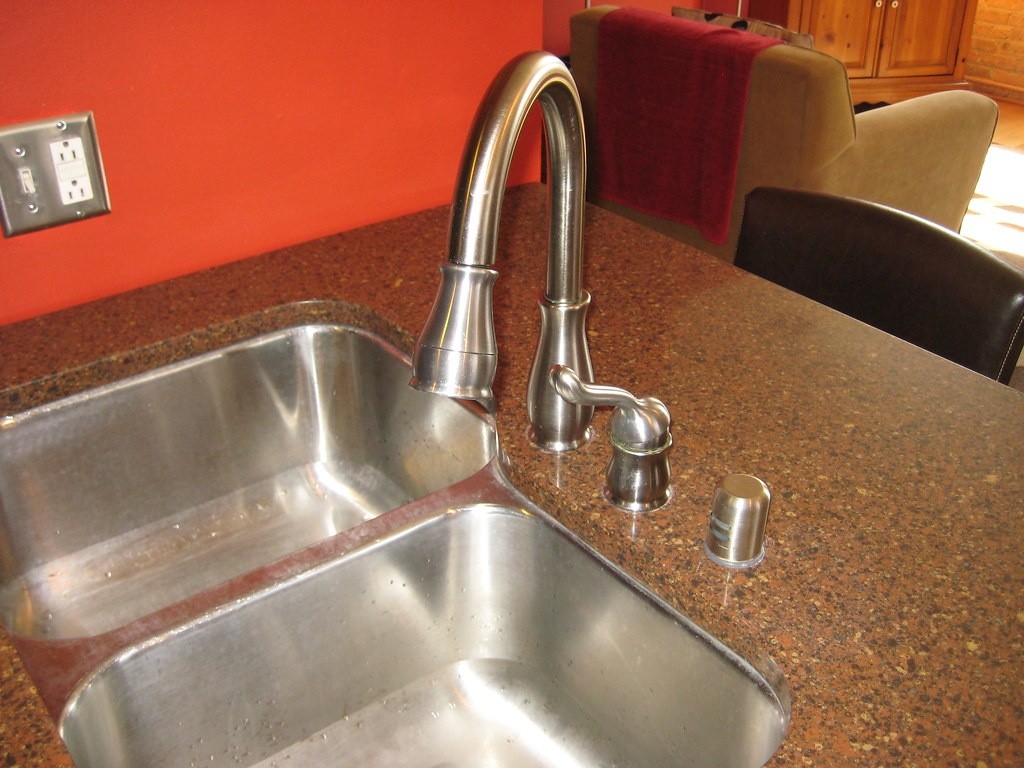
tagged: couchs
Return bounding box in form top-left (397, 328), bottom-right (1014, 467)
top-left (569, 4), bottom-right (1008, 264)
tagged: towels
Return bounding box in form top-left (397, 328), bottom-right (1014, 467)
top-left (585, 5), bottom-right (787, 245)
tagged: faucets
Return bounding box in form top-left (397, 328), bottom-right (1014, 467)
top-left (404, 49), bottom-right (595, 458)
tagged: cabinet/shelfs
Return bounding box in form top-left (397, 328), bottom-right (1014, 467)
top-left (744, 0), bottom-right (981, 105)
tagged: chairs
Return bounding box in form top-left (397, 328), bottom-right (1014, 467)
top-left (725, 181), bottom-right (1024, 382)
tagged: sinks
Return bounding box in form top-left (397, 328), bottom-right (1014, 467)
top-left (29, 456), bottom-right (791, 768)
top-left (0, 295), bottom-right (502, 684)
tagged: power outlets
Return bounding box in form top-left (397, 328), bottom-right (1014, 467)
top-left (0, 109), bottom-right (114, 238)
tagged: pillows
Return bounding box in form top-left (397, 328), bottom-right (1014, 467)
top-left (670, 5), bottom-right (815, 50)
